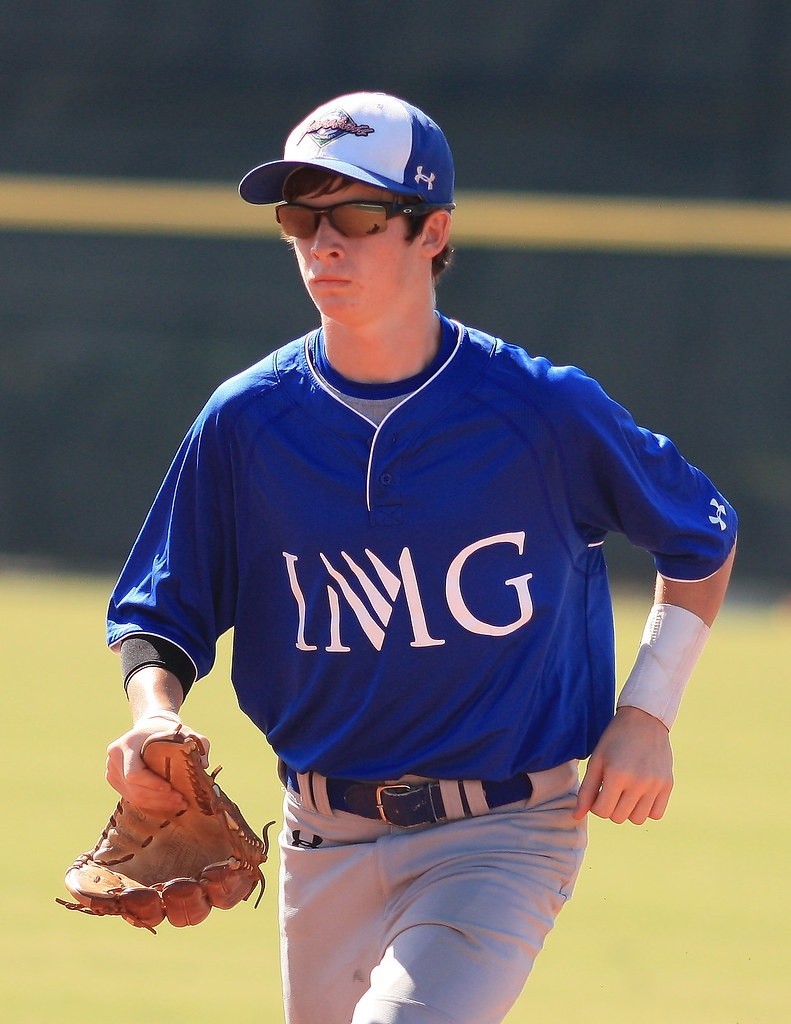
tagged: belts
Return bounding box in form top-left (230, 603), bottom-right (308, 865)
top-left (285, 764), bottom-right (534, 829)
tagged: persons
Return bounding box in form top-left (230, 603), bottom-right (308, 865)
top-left (107, 93), bottom-right (739, 1024)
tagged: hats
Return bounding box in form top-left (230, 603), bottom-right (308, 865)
top-left (237, 91), bottom-right (456, 209)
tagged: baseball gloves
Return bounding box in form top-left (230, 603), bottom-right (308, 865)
top-left (57, 723), bottom-right (276, 934)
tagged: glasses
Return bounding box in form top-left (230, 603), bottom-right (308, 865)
top-left (275, 202), bottom-right (456, 238)
